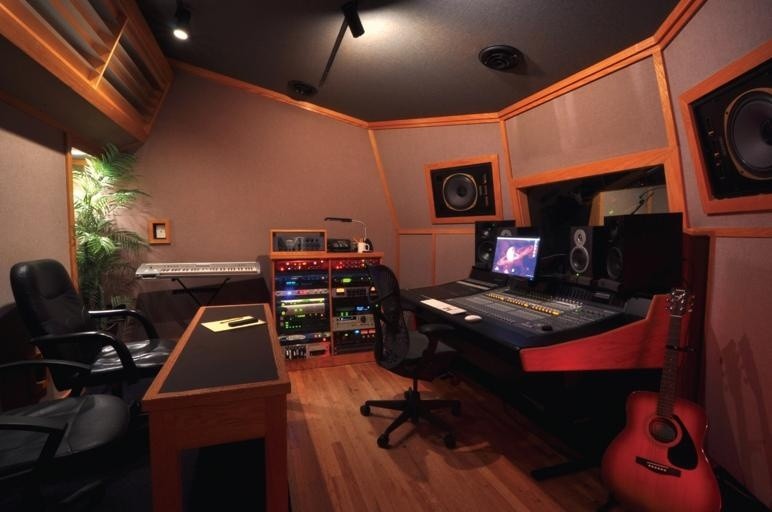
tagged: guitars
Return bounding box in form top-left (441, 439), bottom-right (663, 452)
top-left (600, 286), bottom-right (723, 512)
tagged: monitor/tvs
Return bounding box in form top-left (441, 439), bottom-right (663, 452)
top-left (491, 235), bottom-right (544, 296)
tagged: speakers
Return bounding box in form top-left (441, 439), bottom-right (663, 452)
top-left (430, 162), bottom-right (495, 217)
top-left (474, 221), bottom-right (514, 269)
top-left (604, 213), bottom-right (682, 291)
top-left (687, 58), bottom-right (772, 200)
top-left (570, 226), bottom-right (593, 276)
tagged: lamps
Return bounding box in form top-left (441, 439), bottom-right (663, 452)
top-left (173, 5), bottom-right (191, 41)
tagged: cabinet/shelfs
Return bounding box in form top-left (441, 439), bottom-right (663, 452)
top-left (265, 250), bottom-right (384, 372)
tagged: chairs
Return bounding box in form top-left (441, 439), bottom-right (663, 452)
top-left (359, 263), bottom-right (477, 449)
top-left (0, 358), bottom-right (132, 511)
top-left (8, 257), bottom-right (176, 434)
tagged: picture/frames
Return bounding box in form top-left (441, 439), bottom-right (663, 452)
top-left (148, 216), bottom-right (172, 245)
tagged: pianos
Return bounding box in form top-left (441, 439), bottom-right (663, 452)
top-left (134, 260), bottom-right (262, 280)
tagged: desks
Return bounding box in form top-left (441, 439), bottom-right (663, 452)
top-left (141, 303), bottom-right (293, 512)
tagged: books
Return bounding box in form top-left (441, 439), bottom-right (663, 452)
top-left (202, 315), bottom-right (267, 332)
